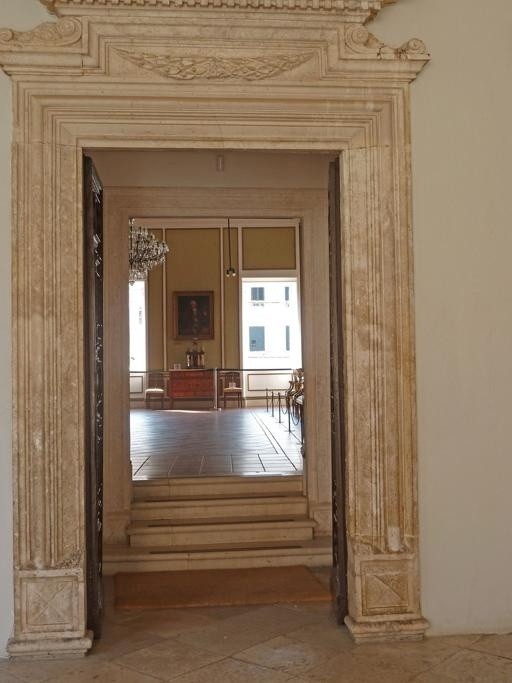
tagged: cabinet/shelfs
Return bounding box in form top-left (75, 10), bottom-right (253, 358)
top-left (168, 369), bottom-right (215, 410)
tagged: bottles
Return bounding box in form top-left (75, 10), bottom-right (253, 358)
top-left (186, 346), bottom-right (207, 368)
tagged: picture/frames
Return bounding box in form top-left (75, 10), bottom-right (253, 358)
top-left (171, 290), bottom-right (215, 340)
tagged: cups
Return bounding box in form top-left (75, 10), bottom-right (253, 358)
top-left (173, 364), bottom-right (180, 370)
top-left (229, 383), bottom-right (236, 388)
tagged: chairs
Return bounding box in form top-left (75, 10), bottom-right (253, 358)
top-left (144, 373), bottom-right (166, 409)
top-left (222, 371), bottom-right (244, 408)
